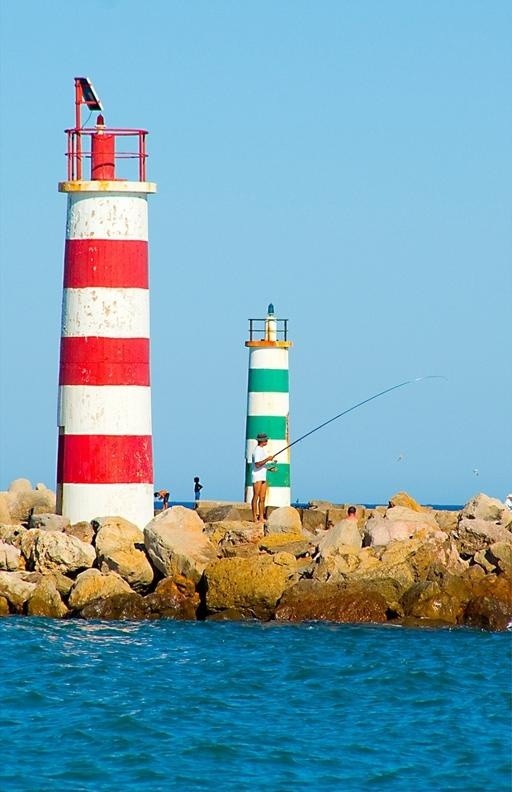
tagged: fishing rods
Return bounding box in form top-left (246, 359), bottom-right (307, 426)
top-left (272, 376), bottom-right (448, 458)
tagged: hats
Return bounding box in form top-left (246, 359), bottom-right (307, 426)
top-left (256, 433), bottom-right (269, 442)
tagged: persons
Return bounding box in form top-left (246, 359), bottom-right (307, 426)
top-left (194, 477), bottom-right (204, 509)
top-left (154, 489), bottom-right (171, 511)
top-left (341, 506), bottom-right (358, 523)
top-left (248, 433), bottom-right (274, 524)
